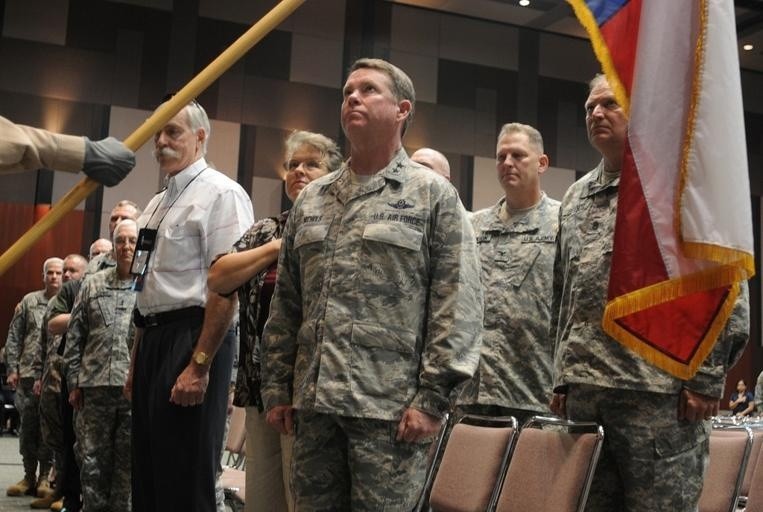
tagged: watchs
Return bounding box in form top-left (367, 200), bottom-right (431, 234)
top-left (190, 352), bottom-right (213, 367)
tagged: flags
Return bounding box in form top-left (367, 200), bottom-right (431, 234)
top-left (567, 1), bottom-right (752, 381)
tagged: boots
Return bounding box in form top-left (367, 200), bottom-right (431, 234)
top-left (49, 496), bottom-right (64, 512)
top-left (30, 488), bottom-right (61, 510)
top-left (6, 473), bottom-right (37, 497)
top-left (36, 473), bottom-right (54, 498)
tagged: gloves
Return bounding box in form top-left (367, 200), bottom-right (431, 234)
top-left (82, 134), bottom-right (136, 188)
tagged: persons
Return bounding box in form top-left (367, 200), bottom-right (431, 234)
top-left (80, 201), bottom-right (141, 279)
top-left (556, 75), bottom-right (747, 512)
top-left (469, 124), bottom-right (571, 417)
top-left (5, 259), bottom-right (61, 498)
top-left (755, 371), bottom-right (762, 417)
top-left (30, 255), bottom-right (89, 510)
top-left (125, 99), bottom-right (254, 512)
top-left (259, 60), bottom-right (485, 512)
top-left (43, 239), bottom-right (115, 331)
top-left (61, 220), bottom-right (138, 512)
top-left (407, 146), bottom-right (449, 181)
top-left (1, 116), bottom-right (136, 188)
top-left (205, 130), bottom-right (350, 512)
top-left (729, 379), bottom-right (753, 417)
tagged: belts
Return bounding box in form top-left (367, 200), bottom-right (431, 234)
top-left (143, 313), bottom-right (161, 329)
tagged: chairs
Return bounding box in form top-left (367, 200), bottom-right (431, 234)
top-left (487, 416), bottom-right (604, 511)
top-left (696, 426), bottom-right (754, 511)
top-left (742, 418), bottom-right (763, 512)
top-left (416, 416), bottom-right (517, 512)
top-left (220, 406), bottom-right (247, 502)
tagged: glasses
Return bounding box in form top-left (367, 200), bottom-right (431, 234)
top-left (285, 157), bottom-right (327, 169)
